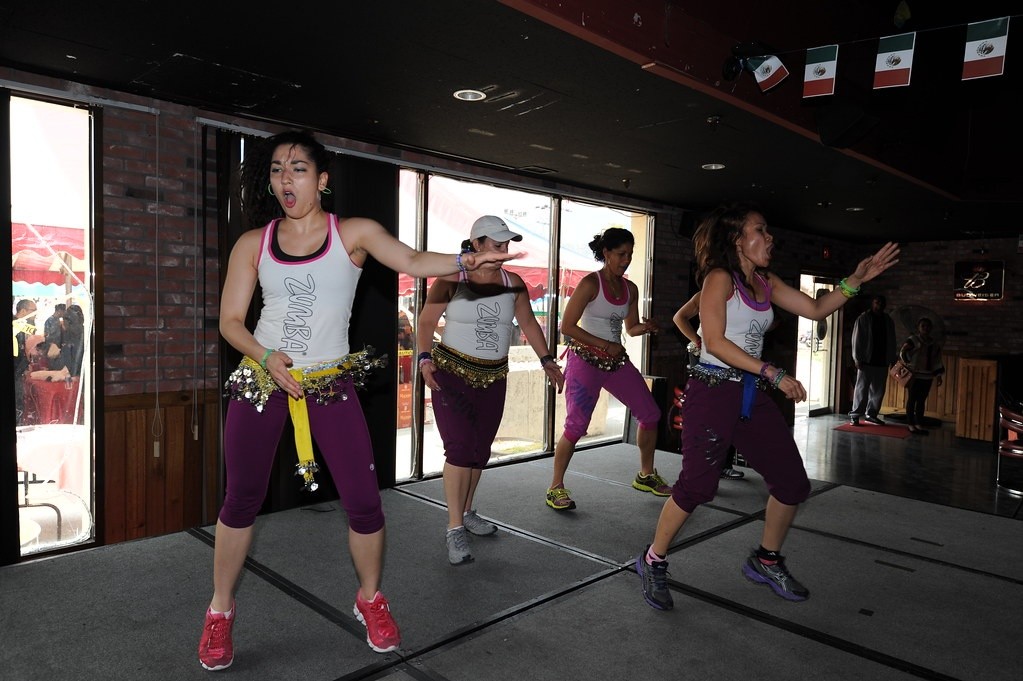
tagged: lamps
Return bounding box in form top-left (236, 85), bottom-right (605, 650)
top-left (820, 244), bottom-right (832, 262)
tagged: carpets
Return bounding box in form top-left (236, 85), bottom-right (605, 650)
top-left (832, 419), bottom-right (913, 439)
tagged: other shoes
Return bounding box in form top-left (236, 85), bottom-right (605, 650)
top-left (865, 416), bottom-right (885, 425)
top-left (908, 423), bottom-right (927, 435)
top-left (850, 416), bottom-right (860, 425)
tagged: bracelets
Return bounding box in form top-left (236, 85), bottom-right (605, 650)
top-left (841, 277), bottom-right (860, 293)
top-left (603, 340), bottom-right (609, 352)
top-left (759, 362), bottom-right (773, 379)
top-left (839, 282), bottom-right (854, 299)
top-left (456, 250), bottom-right (470, 273)
top-left (769, 368), bottom-right (787, 389)
top-left (417, 352), bottom-right (432, 373)
top-left (260, 349), bottom-right (274, 372)
top-left (540, 355), bottom-right (553, 366)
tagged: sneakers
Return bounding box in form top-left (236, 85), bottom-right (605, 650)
top-left (719, 465), bottom-right (745, 480)
top-left (636, 545), bottom-right (675, 610)
top-left (544, 484), bottom-right (575, 511)
top-left (353, 590), bottom-right (403, 653)
top-left (446, 526), bottom-right (476, 567)
top-left (632, 468), bottom-right (673, 496)
top-left (462, 510), bottom-right (498, 536)
top-left (742, 549), bottom-right (811, 602)
top-left (197, 598), bottom-right (237, 670)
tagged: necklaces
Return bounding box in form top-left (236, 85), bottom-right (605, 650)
top-left (606, 270), bottom-right (620, 301)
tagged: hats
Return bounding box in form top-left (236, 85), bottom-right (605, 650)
top-left (470, 215), bottom-right (523, 242)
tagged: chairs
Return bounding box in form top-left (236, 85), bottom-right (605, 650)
top-left (997, 402), bottom-right (1023, 496)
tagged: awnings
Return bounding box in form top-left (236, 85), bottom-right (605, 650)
top-left (11, 223), bottom-right (88, 288)
top-left (399, 260), bottom-right (590, 302)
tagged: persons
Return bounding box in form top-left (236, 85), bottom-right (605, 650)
top-left (11, 299), bottom-right (84, 427)
top-left (847, 296), bottom-right (897, 426)
top-left (198, 131), bottom-right (530, 670)
top-left (545, 228), bottom-right (673, 509)
top-left (397, 310), bottom-right (412, 347)
top-left (634, 200), bottom-right (900, 610)
top-left (900, 318), bottom-right (945, 434)
top-left (417, 216), bottom-right (564, 565)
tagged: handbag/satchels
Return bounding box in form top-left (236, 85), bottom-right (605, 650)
top-left (889, 361), bottom-right (914, 388)
